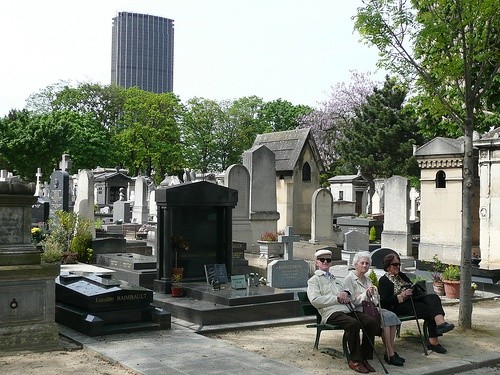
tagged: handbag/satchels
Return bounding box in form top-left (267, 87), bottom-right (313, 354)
top-left (362, 290), bottom-right (382, 336)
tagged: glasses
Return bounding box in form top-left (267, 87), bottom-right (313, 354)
top-left (317, 258), bottom-right (332, 263)
top-left (389, 263), bottom-right (401, 266)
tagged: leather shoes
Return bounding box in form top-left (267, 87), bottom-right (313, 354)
top-left (349, 359), bottom-right (369, 373)
top-left (361, 359), bottom-right (376, 372)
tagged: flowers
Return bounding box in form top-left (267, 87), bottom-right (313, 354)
top-left (423, 253), bottom-right (443, 282)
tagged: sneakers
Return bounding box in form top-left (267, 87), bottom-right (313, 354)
top-left (427, 341), bottom-right (447, 354)
top-left (436, 322), bottom-right (454, 334)
top-left (385, 354), bottom-right (403, 366)
top-left (384, 351), bottom-right (405, 363)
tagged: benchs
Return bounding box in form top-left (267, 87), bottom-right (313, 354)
top-left (298, 279), bottom-right (426, 362)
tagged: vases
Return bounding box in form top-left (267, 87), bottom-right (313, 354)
top-left (433, 280), bottom-right (445, 296)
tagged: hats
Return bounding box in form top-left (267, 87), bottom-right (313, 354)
top-left (315, 250), bottom-right (332, 258)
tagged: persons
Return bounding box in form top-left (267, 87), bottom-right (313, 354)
top-left (379, 253), bottom-right (456, 354)
top-left (344, 251), bottom-right (406, 366)
top-left (307, 250), bottom-right (376, 374)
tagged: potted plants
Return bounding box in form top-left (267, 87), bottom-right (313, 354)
top-left (443, 265), bottom-right (463, 300)
top-left (172, 283), bottom-right (184, 297)
top-left (172, 233), bottom-right (189, 282)
top-left (471, 252), bottom-right (481, 266)
top-left (257, 233), bottom-right (284, 259)
